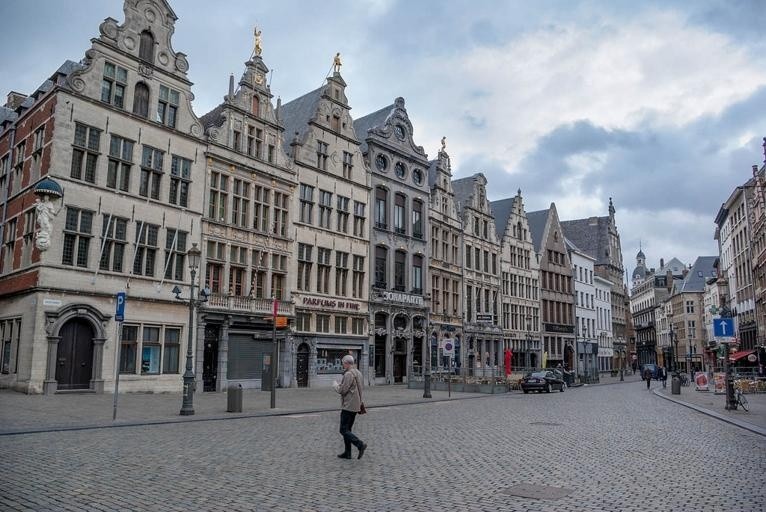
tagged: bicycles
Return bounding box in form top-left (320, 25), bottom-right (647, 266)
top-left (727, 386), bottom-right (751, 411)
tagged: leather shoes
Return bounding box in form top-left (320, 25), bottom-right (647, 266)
top-left (338, 453), bottom-right (352, 459)
top-left (357, 444), bottom-right (367, 459)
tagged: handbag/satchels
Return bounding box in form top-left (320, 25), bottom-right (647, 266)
top-left (357, 402), bottom-right (366, 414)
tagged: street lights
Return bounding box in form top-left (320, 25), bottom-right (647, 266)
top-left (413, 292), bottom-right (441, 398)
top-left (686, 334), bottom-right (697, 382)
top-left (668, 323), bottom-right (679, 370)
top-left (577, 325), bottom-right (591, 384)
top-left (613, 337), bottom-right (628, 382)
top-left (172, 242), bottom-right (211, 416)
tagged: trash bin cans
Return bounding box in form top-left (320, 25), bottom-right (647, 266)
top-left (672, 377), bottom-right (680, 394)
top-left (227, 382), bottom-right (243, 412)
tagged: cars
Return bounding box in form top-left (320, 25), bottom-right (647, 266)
top-left (520, 369), bottom-right (566, 394)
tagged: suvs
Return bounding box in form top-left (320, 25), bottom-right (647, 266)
top-left (640, 363), bottom-right (663, 381)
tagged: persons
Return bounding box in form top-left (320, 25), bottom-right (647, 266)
top-left (37, 195), bottom-right (53, 234)
top-left (643, 367), bottom-right (652, 389)
top-left (31, 198), bottom-right (42, 214)
top-left (632, 362), bottom-right (637, 374)
top-left (556, 363), bottom-right (564, 379)
top-left (690, 366), bottom-right (696, 382)
top-left (659, 366), bottom-right (667, 388)
top-left (332, 354), bottom-right (368, 459)
